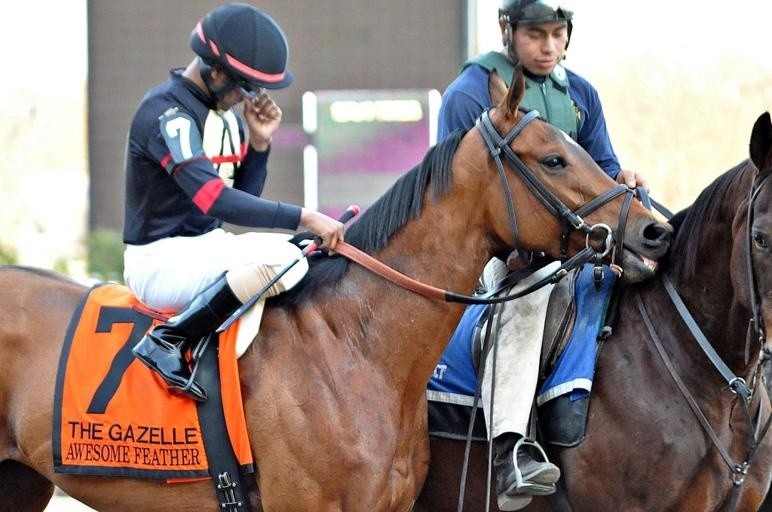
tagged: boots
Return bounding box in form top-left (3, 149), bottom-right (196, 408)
top-left (131, 271), bottom-right (244, 404)
top-left (492, 433), bottom-right (558, 510)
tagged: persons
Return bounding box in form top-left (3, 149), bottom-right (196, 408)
top-left (431, 0), bottom-right (652, 510)
top-left (119, 3), bottom-right (345, 404)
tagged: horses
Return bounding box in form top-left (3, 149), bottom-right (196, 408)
top-left (414, 110), bottom-right (772, 512)
top-left (0, 61), bottom-right (674, 511)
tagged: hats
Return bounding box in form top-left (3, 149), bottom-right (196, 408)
top-left (188, 1), bottom-right (295, 90)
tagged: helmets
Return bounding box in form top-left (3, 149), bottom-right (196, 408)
top-left (498, 0), bottom-right (574, 27)
